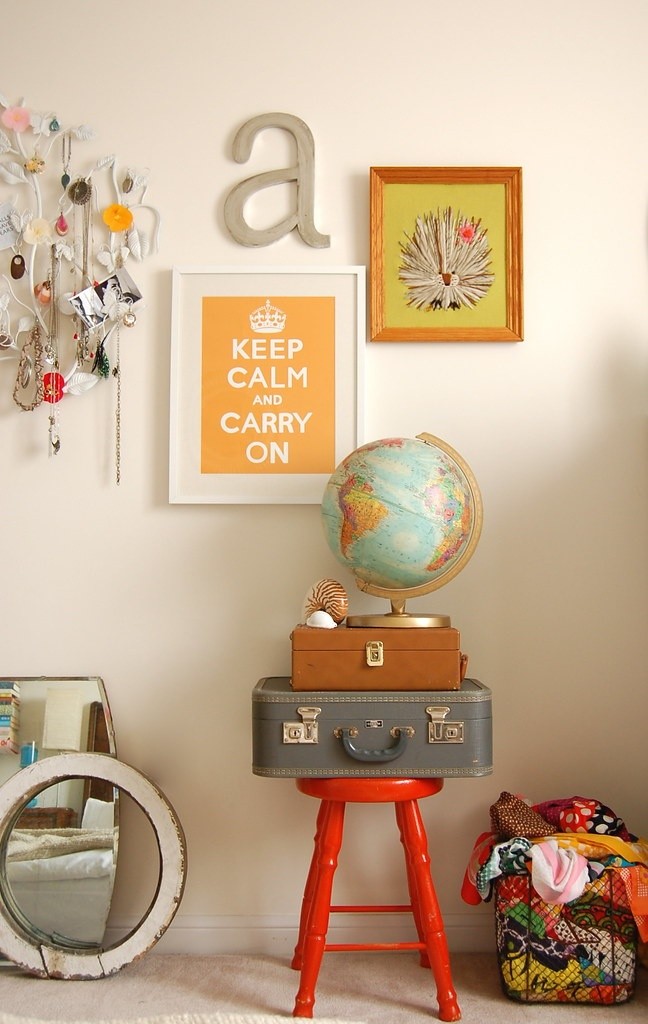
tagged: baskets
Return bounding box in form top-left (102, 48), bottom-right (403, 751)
top-left (493, 870), bottom-right (637, 1007)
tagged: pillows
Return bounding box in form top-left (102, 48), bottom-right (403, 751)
top-left (80, 798), bottom-right (115, 828)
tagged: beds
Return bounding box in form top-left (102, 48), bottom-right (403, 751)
top-left (4, 826), bottom-right (120, 945)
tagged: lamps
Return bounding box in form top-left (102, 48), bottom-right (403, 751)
top-left (42, 701), bottom-right (83, 808)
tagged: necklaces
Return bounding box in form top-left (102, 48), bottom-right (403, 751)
top-left (112, 300), bottom-right (122, 485)
top-left (7, 244), bottom-right (102, 457)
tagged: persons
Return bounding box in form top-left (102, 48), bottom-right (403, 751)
top-left (71, 298), bottom-right (104, 328)
top-left (101, 276), bottom-right (139, 316)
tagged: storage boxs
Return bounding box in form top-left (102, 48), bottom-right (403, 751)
top-left (493, 869), bottom-right (637, 1004)
top-left (290, 623), bottom-right (468, 691)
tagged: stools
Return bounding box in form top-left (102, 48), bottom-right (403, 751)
top-left (289, 776), bottom-right (461, 1022)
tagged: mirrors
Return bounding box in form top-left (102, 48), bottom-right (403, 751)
top-left (0, 676), bottom-right (121, 946)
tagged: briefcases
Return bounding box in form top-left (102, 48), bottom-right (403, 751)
top-left (251, 677), bottom-right (491, 779)
top-left (289, 623), bottom-right (469, 691)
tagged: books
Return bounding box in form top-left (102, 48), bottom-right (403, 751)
top-left (0, 681), bottom-right (21, 755)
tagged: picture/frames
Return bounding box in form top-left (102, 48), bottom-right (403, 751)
top-left (167, 264), bottom-right (366, 505)
top-left (369, 166), bottom-right (525, 341)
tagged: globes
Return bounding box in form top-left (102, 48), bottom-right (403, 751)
top-left (320, 432), bottom-right (484, 628)
